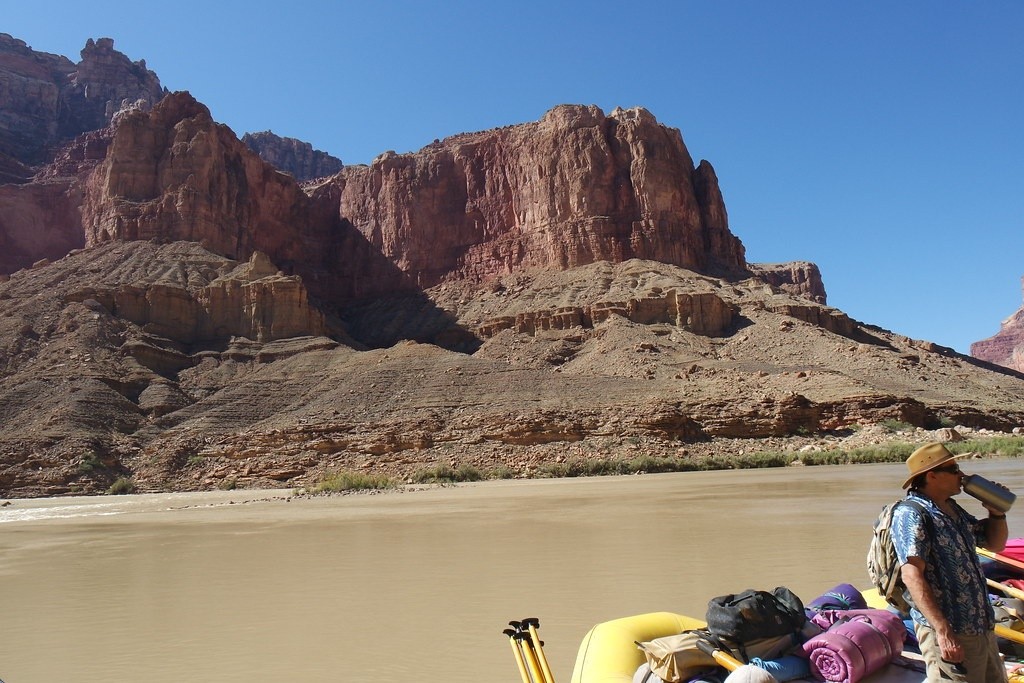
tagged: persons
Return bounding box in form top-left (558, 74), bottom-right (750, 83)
top-left (889, 441), bottom-right (1009, 683)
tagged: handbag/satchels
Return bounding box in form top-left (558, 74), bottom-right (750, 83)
top-left (633, 631), bottom-right (723, 683)
top-left (705, 586), bottom-right (807, 665)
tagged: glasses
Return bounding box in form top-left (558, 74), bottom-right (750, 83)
top-left (936, 464), bottom-right (959, 474)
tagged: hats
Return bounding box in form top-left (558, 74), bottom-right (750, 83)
top-left (901, 441), bottom-right (972, 489)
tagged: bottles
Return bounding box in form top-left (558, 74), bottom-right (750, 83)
top-left (961, 474), bottom-right (1016, 512)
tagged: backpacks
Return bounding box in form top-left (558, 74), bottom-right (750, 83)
top-left (866, 499), bottom-right (937, 616)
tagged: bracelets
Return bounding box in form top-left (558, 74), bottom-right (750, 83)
top-left (989, 514), bottom-right (1006, 519)
